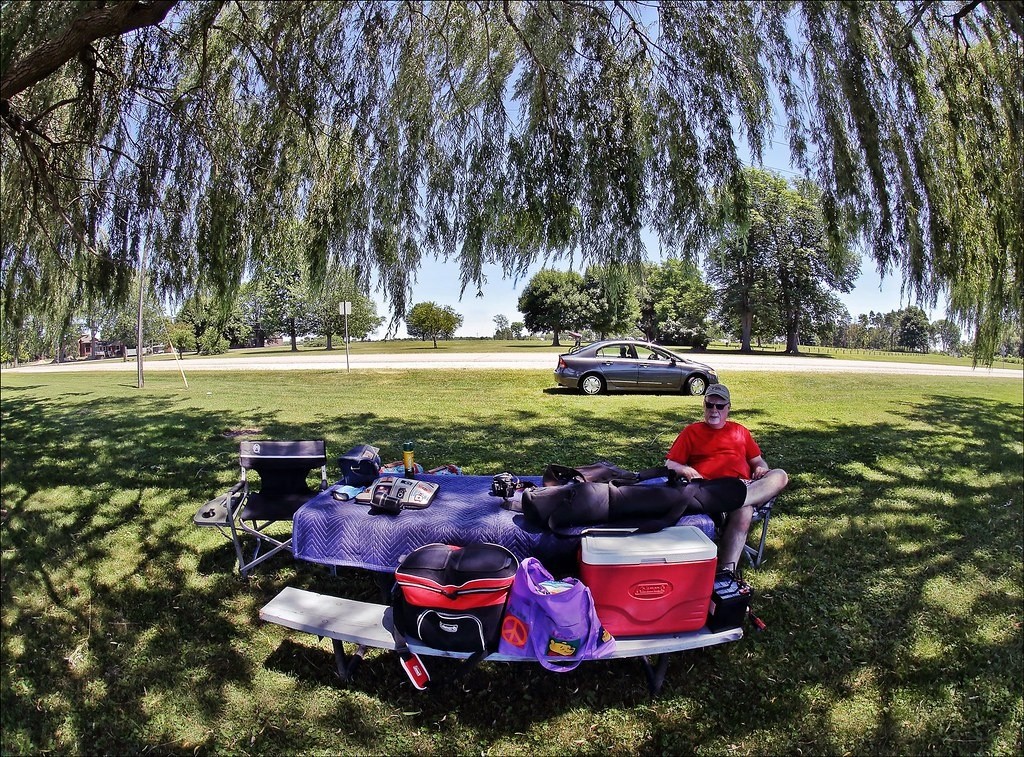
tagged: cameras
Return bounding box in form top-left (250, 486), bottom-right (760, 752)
top-left (491, 472), bottom-right (518, 498)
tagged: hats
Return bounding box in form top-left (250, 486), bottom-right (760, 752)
top-left (705, 384), bottom-right (730, 401)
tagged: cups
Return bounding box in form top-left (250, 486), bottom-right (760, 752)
top-left (402, 440), bottom-right (414, 479)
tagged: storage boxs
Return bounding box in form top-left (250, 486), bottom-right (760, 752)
top-left (577, 525), bottom-right (755, 637)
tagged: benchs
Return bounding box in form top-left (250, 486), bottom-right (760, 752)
top-left (258, 586), bottom-right (744, 701)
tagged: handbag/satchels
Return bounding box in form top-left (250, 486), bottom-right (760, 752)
top-left (390, 544), bottom-right (518, 653)
top-left (337, 443), bottom-right (381, 486)
top-left (498, 555), bottom-right (615, 673)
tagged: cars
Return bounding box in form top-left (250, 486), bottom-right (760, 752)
top-left (554, 339), bottom-right (718, 396)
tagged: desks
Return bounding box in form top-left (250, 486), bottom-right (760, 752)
top-left (291, 470), bottom-right (716, 607)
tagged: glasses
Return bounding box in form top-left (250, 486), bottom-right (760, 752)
top-left (705, 399), bottom-right (730, 410)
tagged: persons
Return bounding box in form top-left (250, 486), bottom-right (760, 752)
top-left (664, 383), bottom-right (789, 575)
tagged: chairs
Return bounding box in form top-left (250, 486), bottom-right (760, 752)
top-left (226, 439), bottom-right (330, 583)
top-left (617, 348), bottom-right (632, 357)
